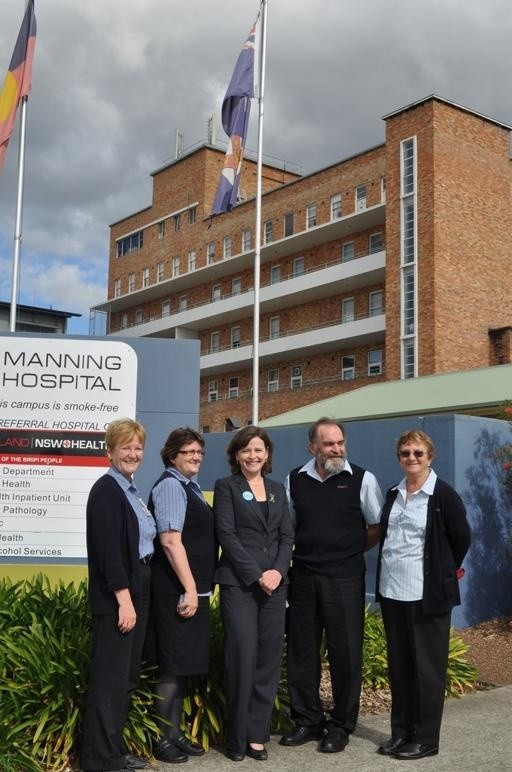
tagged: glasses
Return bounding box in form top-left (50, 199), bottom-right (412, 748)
top-left (173, 448), bottom-right (205, 458)
top-left (396, 449), bottom-right (432, 457)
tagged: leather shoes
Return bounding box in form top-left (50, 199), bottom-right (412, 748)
top-left (378, 735), bottom-right (406, 755)
top-left (152, 737), bottom-right (188, 762)
top-left (319, 724), bottom-right (349, 754)
top-left (122, 750), bottom-right (146, 768)
top-left (393, 738), bottom-right (440, 759)
top-left (226, 750), bottom-right (246, 761)
top-left (247, 743), bottom-right (270, 763)
top-left (167, 729), bottom-right (206, 756)
top-left (278, 720), bottom-right (324, 745)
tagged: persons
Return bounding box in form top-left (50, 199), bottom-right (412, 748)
top-left (213, 427), bottom-right (296, 761)
top-left (375, 429), bottom-right (470, 759)
top-left (147, 427), bottom-right (219, 763)
top-left (284, 417), bottom-right (385, 753)
top-left (79, 418), bottom-right (157, 772)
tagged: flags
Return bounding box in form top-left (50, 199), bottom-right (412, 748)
top-left (210, 5), bottom-right (263, 216)
top-left (0, 0), bottom-right (37, 172)
